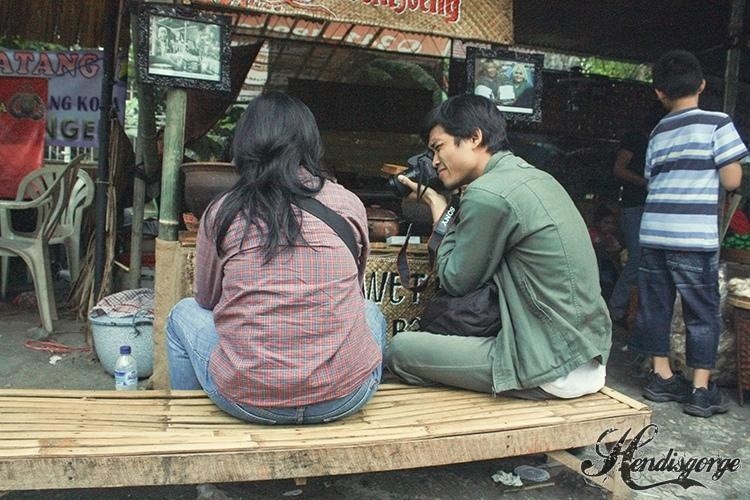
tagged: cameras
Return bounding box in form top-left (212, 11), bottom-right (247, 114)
top-left (388, 150), bottom-right (442, 201)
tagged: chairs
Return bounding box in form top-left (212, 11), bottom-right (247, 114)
top-left (0, 154), bottom-right (96, 339)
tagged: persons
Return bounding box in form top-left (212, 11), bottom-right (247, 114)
top-left (158, 27), bottom-right (167, 54)
top-left (156, 129), bottom-right (164, 155)
top-left (588, 101), bottom-right (669, 329)
top-left (477, 60), bottom-right (533, 98)
top-left (387, 93), bottom-right (612, 401)
top-left (635, 49), bottom-right (750, 417)
top-left (166, 91), bottom-right (386, 424)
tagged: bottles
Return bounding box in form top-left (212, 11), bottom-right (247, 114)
top-left (114, 345), bottom-right (138, 391)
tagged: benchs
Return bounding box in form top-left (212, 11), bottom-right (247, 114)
top-left (1, 387), bottom-right (653, 500)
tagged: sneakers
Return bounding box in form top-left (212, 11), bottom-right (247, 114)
top-left (643, 368), bottom-right (689, 402)
top-left (685, 379), bottom-right (729, 416)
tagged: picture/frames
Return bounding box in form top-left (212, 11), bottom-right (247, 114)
top-left (467, 44), bottom-right (544, 125)
top-left (139, 2), bottom-right (233, 90)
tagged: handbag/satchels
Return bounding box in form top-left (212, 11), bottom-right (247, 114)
top-left (419, 279), bottom-right (501, 338)
